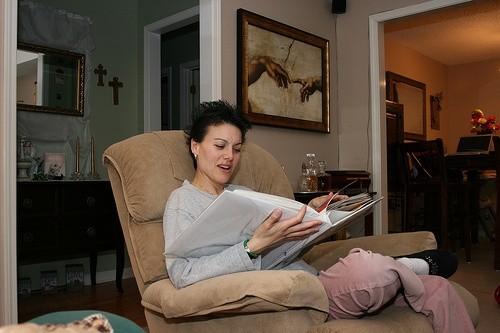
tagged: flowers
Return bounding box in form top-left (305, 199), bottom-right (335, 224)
top-left (470, 107), bottom-right (500, 133)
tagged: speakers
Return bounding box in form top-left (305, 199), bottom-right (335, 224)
top-left (331, 0), bottom-right (347, 14)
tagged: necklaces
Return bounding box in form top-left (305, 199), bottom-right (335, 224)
top-left (192, 180), bottom-right (212, 195)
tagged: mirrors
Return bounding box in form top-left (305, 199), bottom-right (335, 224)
top-left (386, 70), bottom-right (427, 140)
top-left (17, 41), bottom-right (86, 117)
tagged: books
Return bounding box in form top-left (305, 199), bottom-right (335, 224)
top-left (40, 269), bottom-right (58, 295)
top-left (65, 263), bottom-right (84, 293)
top-left (163, 180), bottom-right (384, 269)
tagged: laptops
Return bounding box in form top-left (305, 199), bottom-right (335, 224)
top-left (446, 134), bottom-right (494, 157)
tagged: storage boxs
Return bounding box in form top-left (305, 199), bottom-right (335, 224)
top-left (17, 264), bottom-right (84, 299)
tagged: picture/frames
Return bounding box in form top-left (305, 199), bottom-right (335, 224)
top-left (237, 8), bottom-right (331, 134)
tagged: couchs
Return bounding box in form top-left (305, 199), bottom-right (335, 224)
top-left (99, 128), bottom-right (480, 333)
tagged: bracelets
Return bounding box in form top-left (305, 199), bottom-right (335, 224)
top-left (244, 239), bottom-right (258, 258)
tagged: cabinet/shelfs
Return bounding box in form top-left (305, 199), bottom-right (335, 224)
top-left (386, 101), bottom-right (408, 235)
top-left (17, 180), bottom-right (126, 293)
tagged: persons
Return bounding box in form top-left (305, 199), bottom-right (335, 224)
top-left (163, 100), bottom-right (476, 333)
top-left (17, 278), bottom-right (32, 296)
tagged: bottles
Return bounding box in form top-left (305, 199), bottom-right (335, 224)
top-left (296, 153), bottom-right (331, 193)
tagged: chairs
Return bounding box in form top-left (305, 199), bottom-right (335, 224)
top-left (399, 137), bottom-right (476, 264)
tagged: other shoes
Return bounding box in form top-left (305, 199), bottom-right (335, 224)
top-left (392, 246), bottom-right (460, 278)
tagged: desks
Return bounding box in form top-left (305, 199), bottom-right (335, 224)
top-left (293, 191), bottom-right (377, 236)
top-left (424, 152), bottom-right (500, 271)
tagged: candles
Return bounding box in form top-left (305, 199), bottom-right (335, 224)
top-left (75, 136), bottom-right (79, 172)
top-left (91, 136), bottom-right (95, 174)
